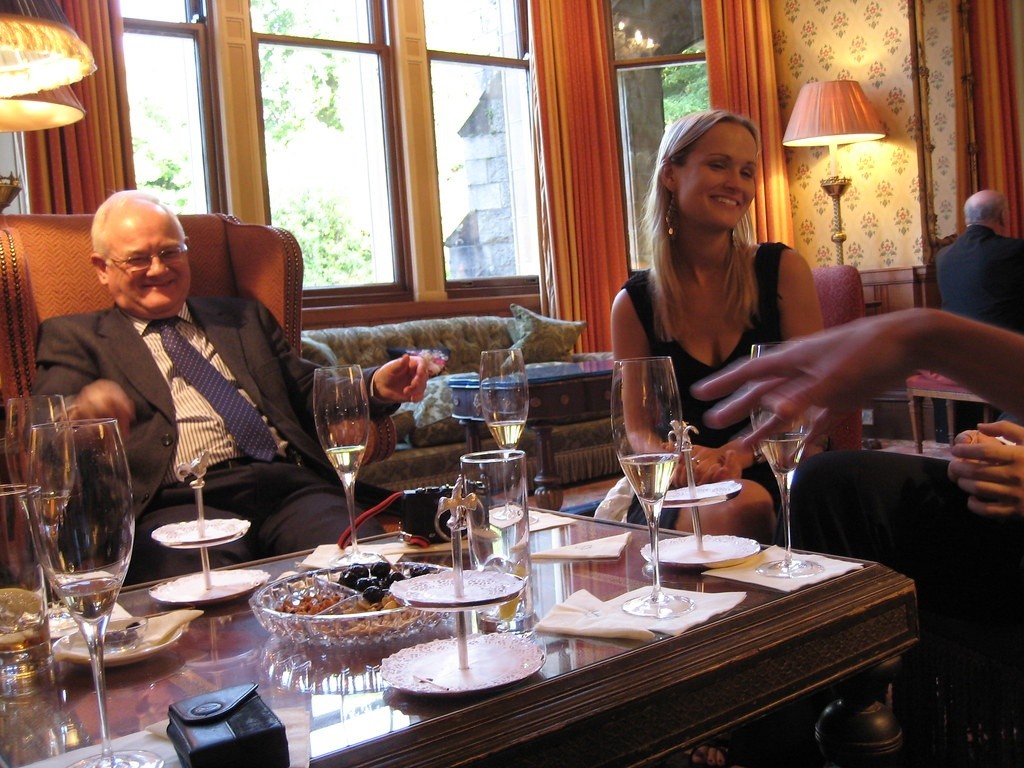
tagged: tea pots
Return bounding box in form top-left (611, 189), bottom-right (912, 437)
top-left (610, 354), bottom-right (695, 618)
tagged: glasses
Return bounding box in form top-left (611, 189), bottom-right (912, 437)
top-left (105, 246), bottom-right (185, 272)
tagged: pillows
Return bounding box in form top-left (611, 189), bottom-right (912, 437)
top-left (506, 301), bottom-right (587, 362)
top-left (387, 346), bottom-right (453, 380)
top-left (495, 319), bottom-right (523, 370)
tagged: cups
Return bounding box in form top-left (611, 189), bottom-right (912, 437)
top-left (461, 448), bottom-right (536, 634)
top-left (0, 483), bottom-right (55, 675)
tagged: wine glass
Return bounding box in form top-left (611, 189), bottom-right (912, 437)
top-left (750, 341), bottom-right (825, 578)
top-left (3, 394), bottom-right (68, 608)
top-left (27, 417), bottom-right (166, 767)
top-left (478, 348), bottom-right (541, 526)
top-left (312, 363), bottom-right (382, 565)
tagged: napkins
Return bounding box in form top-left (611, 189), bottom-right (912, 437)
top-left (47, 599), bottom-right (132, 637)
top-left (292, 507), bottom-right (866, 641)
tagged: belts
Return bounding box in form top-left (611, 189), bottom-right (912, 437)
top-left (207, 455), bottom-right (295, 472)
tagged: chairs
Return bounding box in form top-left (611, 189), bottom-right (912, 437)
top-left (907, 265), bottom-right (994, 456)
top-left (0, 213), bottom-right (305, 590)
top-left (802, 262), bottom-right (865, 449)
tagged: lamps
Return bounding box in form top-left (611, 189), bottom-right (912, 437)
top-left (0, 0), bottom-right (97, 214)
top-left (781, 79), bottom-right (888, 266)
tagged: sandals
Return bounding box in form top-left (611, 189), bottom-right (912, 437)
top-left (688, 738), bottom-right (730, 768)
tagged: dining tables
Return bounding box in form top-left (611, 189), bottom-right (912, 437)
top-left (0, 506), bottom-right (919, 767)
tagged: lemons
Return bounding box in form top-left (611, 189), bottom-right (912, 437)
top-left (499, 566), bottom-right (527, 619)
top-left (0, 588), bottom-right (42, 614)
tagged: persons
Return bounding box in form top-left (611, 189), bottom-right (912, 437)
top-left (594, 110), bottom-right (864, 550)
top-left (29, 189), bottom-right (426, 584)
top-left (689, 307), bottom-right (1024, 768)
top-left (932, 190), bottom-right (1023, 336)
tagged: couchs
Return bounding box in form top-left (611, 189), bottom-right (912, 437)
top-left (292, 312), bottom-right (618, 493)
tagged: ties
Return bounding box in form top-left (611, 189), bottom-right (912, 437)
top-left (148, 315), bottom-right (279, 462)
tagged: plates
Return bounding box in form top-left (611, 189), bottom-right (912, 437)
top-left (52, 615), bottom-right (184, 668)
top-left (250, 559), bottom-right (464, 643)
top-left (640, 535), bottom-right (759, 569)
top-left (260, 618), bottom-right (459, 695)
top-left (662, 481), bottom-right (742, 508)
